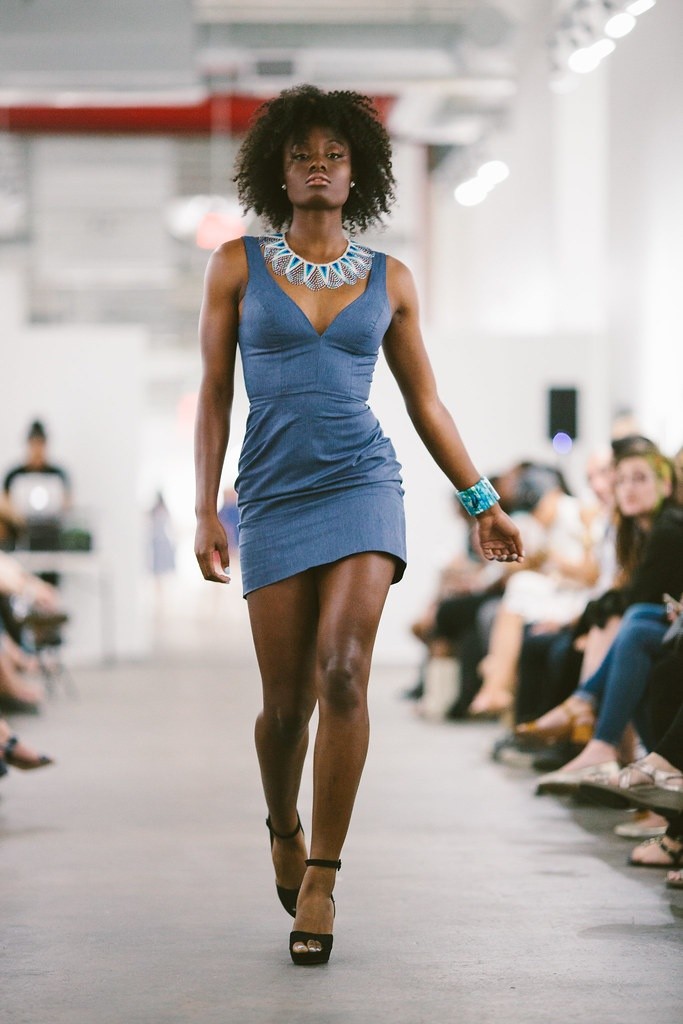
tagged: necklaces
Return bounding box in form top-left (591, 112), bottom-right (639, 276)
top-left (260, 224), bottom-right (377, 290)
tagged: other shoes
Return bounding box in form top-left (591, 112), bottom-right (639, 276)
top-left (628, 834), bottom-right (683, 869)
top-left (536, 761), bottom-right (620, 793)
top-left (613, 815), bottom-right (668, 836)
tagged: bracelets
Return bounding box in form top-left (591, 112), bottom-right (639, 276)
top-left (456, 475), bottom-right (501, 517)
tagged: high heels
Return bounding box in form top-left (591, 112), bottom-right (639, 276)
top-left (266, 809), bottom-right (309, 918)
top-left (291, 860), bottom-right (342, 966)
top-left (0, 734), bottom-right (52, 777)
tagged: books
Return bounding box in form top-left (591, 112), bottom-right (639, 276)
top-left (403, 432), bottom-right (683, 890)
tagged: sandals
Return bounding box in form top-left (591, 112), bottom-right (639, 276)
top-left (516, 703), bottom-right (595, 744)
top-left (665, 869), bottom-right (683, 888)
top-left (581, 760), bottom-right (683, 817)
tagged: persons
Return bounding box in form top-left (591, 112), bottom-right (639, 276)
top-left (4, 419), bottom-right (75, 657)
top-left (214, 486), bottom-right (241, 571)
top-left (146, 489), bottom-right (180, 618)
top-left (188, 86), bottom-right (524, 965)
top-left (0, 493), bottom-right (67, 775)
